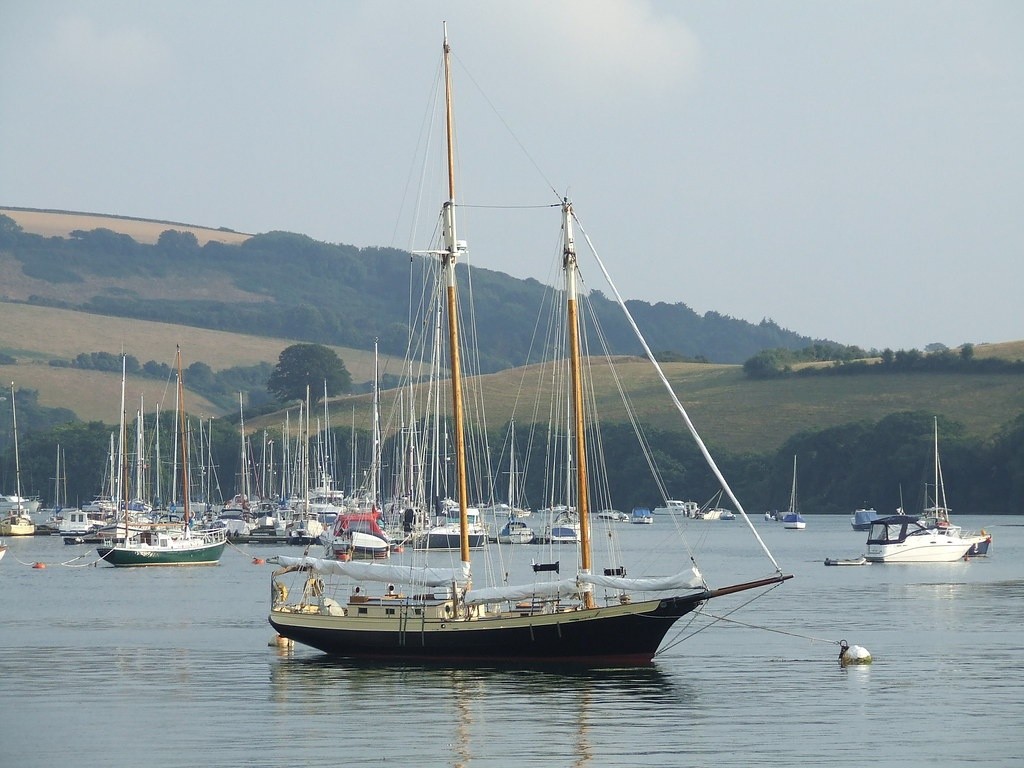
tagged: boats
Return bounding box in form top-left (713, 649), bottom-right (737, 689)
top-left (850, 508), bottom-right (880, 530)
top-left (652, 497), bottom-right (685, 515)
top-left (682, 499), bottom-right (701, 518)
top-left (630, 507), bottom-right (654, 524)
top-left (0, 492), bottom-right (40, 514)
top-left (537, 504), bottom-right (576, 513)
top-left (598, 510), bottom-right (629, 523)
top-left (695, 507), bottom-right (736, 520)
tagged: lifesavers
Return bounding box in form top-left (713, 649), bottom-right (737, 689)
top-left (304, 578), bottom-right (322, 597)
top-left (278, 583), bottom-right (288, 601)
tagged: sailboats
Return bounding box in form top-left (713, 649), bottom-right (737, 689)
top-left (96, 343), bottom-right (228, 567)
top-left (59, 510), bottom-right (109, 535)
top-left (82, 337), bottom-right (591, 560)
top-left (782, 455), bottom-right (806, 529)
top-left (0, 381), bottom-right (36, 536)
top-left (865, 416), bottom-right (992, 562)
top-left (43, 444), bottom-right (66, 533)
top-left (921, 482), bottom-right (952, 524)
top-left (267, 18), bottom-right (794, 670)
